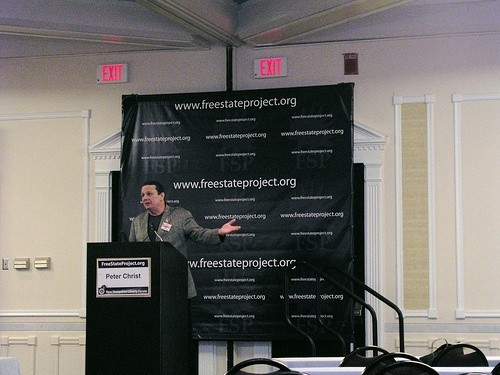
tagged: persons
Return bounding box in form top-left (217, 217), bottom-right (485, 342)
top-left (128, 181), bottom-right (242, 351)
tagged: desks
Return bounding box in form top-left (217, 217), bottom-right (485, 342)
top-left (287, 366), bottom-right (500, 374)
top-left (269, 353), bottom-right (500, 366)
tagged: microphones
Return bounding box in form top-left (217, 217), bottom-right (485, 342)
top-left (149, 222), bottom-right (164, 242)
